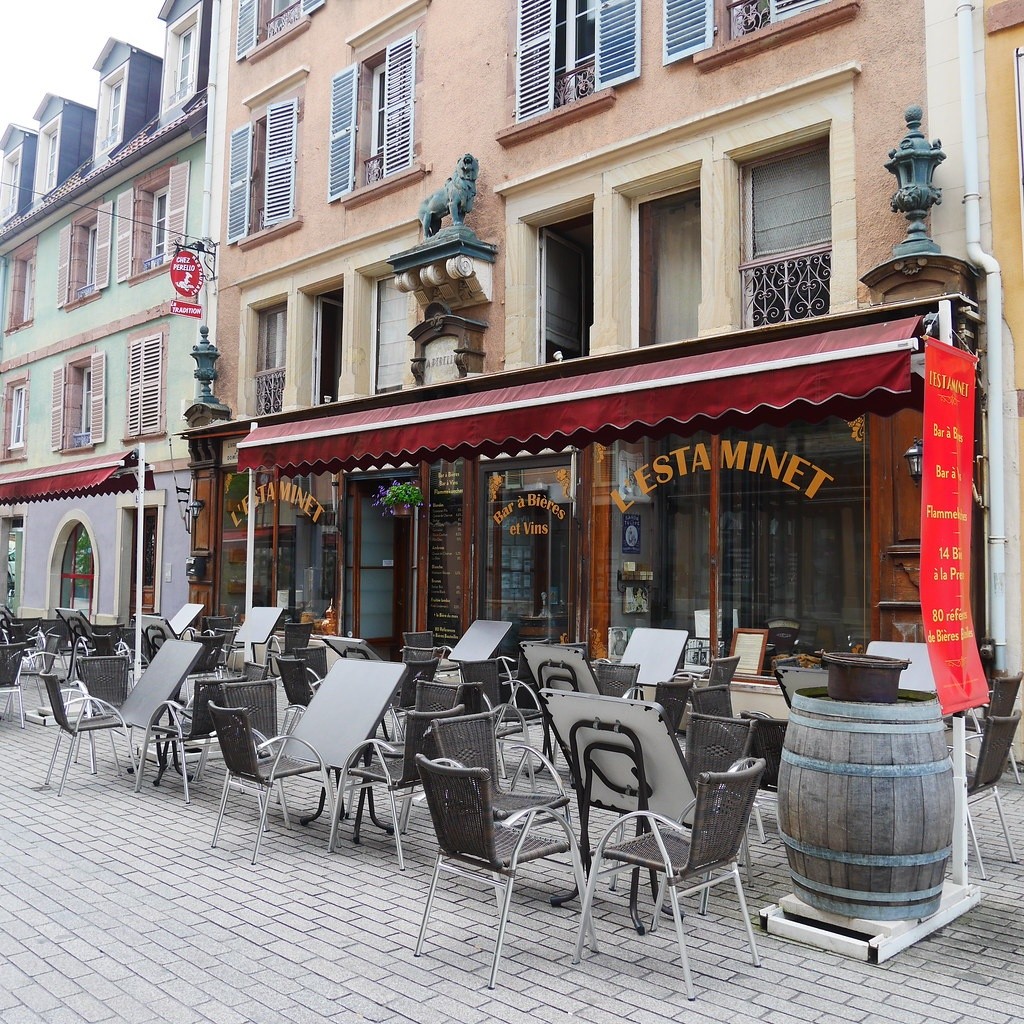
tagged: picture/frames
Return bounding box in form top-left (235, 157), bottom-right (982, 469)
top-left (729, 627), bottom-right (769, 676)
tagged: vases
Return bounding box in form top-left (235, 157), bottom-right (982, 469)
top-left (392, 503), bottom-right (413, 518)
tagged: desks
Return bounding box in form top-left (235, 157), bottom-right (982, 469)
top-left (115, 638), bottom-right (206, 787)
top-left (282, 657), bottom-right (411, 845)
top-left (619, 627), bottom-right (690, 700)
top-left (865, 641), bottom-right (937, 693)
top-left (233, 606), bottom-right (284, 678)
top-left (447, 620), bottom-right (514, 663)
top-left (538, 687), bottom-right (698, 937)
top-left (519, 641), bottom-right (602, 778)
top-left (321, 635), bottom-right (391, 741)
top-left (55, 607), bottom-right (97, 687)
top-left (170, 603), bottom-right (205, 640)
top-left (132, 613), bottom-right (186, 706)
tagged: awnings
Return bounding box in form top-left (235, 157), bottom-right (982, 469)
top-left (237, 315), bottom-right (927, 473)
top-left (0, 450), bottom-right (132, 500)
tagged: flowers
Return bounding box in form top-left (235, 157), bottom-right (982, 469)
top-left (370, 479), bottom-right (424, 519)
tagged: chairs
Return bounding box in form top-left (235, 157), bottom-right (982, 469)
top-left (688, 684), bottom-right (773, 844)
top-left (654, 674), bottom-right (694, 733)
top-left (388, 657), bottom-right (440, 742)
top-left (344, 680), bottom-right (464, 835)
top-left (397, 646), bottom-right (434, 696)
top-left (402, 631), bottom-right (460, 672)
top-left (970, 670), bottom-right (1024, 786)
top-left (413, 754), bottom-right (600, 990)
top-left (498, 652), bottom-right (534, 689)
top-left (459, 659), bottom-right (544, 751)
top-left (594, 662), bottom-right (646, 699)
top-left (0, 616), bottom-right (340, 866)
top-left (946, 709), bottom-right (1022, 881)
top-left (571, 758), bottom-right (767, 1001)
top-left (659, 656), bottom-right (741, 713)
top-left (430, 711), bottom-right (571, 922)
top-left (327, 704), bottom-right (466, 871)
top-left (740, 709), bottom-right (788, 792)
top-left (608, 712), bottom-right (758, 916)
top-left (459, 682), bottom-right (523, 779)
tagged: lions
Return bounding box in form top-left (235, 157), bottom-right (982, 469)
top-left (413, 152), bottom-right (479, 239)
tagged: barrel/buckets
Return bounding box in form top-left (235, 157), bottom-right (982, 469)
top-left (814, 648), bottom-right (912, 703)
top-left (778, 684), bottom-right (955, 921)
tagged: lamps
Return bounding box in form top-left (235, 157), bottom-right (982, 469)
top-left (902, 436), bottom-right (923, 488)
top-left (189, 499), bottom-right (205, 520)
